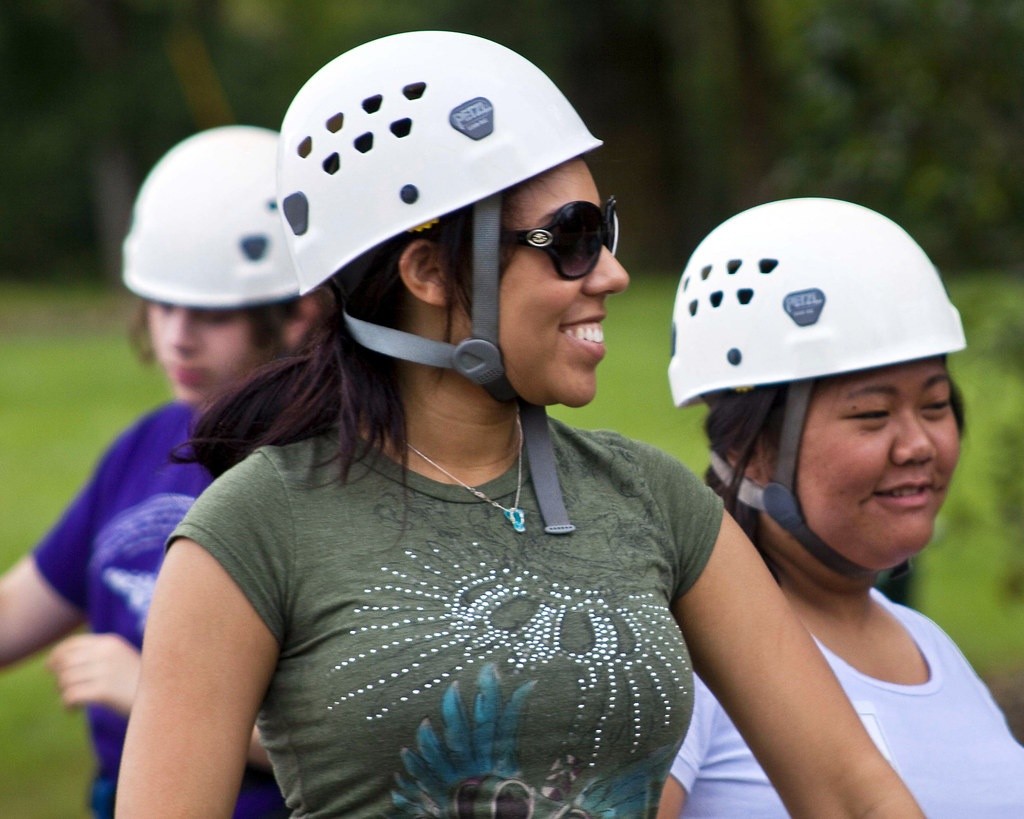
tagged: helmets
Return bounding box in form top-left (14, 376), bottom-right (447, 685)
top-left (666, 198), bottom-right (968, 408)
top-left (276, 31), bottom-right (603, 297)
top-left (119, 124), bottom-right (299, 311)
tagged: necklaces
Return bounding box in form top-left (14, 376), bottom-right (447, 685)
top-left (389, 411), bottom-right (527, 533)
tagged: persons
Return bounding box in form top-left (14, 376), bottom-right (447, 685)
top-left (657, 198), bottom-right (1024, 819)
top-left (0, 125), bottom-right (333, 819)
top-left (113, 27), bottom-right (930, 819)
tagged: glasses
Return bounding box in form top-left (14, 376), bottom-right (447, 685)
top-left (461, 195), bottom-right (620, 281)
